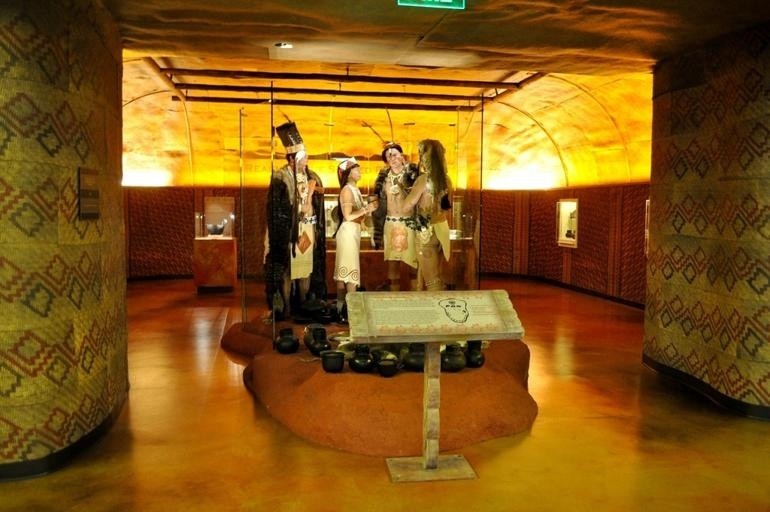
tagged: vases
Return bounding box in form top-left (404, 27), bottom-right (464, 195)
top-left (276, 322), bottom-right (488, 375)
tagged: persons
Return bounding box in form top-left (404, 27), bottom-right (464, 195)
top-left (264, 147), bottom-right (328, 320)
top-left (394, 137), bottom-right (454, 290)
top-left (331, 156), bottom-right (379, 320)
top-left (371, 145), bottom-right (419, 293)
